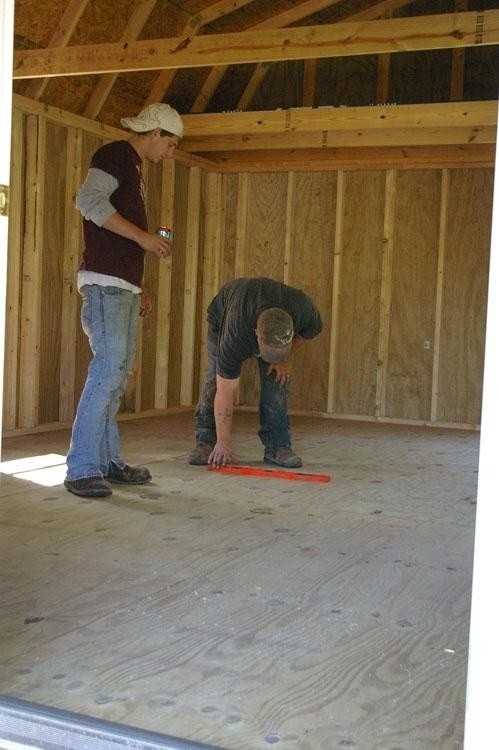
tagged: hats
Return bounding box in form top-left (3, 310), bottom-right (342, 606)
top-left (257, 307), bottom-right (295, 364)
top-left (120, 102), bottom-right (184, 139)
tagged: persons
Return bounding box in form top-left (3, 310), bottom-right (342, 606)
top-left (188, 276), bottom-right (324, 468)
top-left (63, 103), bottom-right (186, 497)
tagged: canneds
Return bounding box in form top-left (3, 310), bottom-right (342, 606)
top-left (156, 226), bottom-right (171, 239)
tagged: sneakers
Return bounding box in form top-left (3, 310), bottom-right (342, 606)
top-left (103, 461), bottom-right (152, 485)
top-left (64, 476), bottom-right (113, 497)
top-left (264, 447), bottom-right (302, 468)
top-left (188, 443), bottom-right (213, 465)
